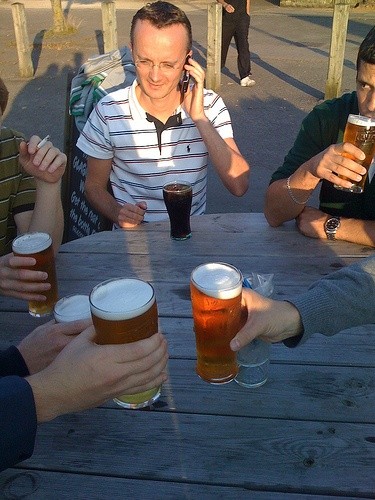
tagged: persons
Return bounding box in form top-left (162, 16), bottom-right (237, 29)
top-left (216, 0), bottom-right (256, 87)
top-left (262, 26), bottom-right (375, 248)
top-left (75, 1), bottom-right (250, 227)
top-left (0, 77), bottom-right (67, 303)
top-left (229, 254), bottom-right (375, 385)
top-left (0, 318), bottom-right (170, 473)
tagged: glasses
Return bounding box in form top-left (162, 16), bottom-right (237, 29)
top-left (132, 44), bottom-right (188, 72)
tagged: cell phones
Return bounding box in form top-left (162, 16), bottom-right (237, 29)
top-left (181, 54), bottom-right (191, 93)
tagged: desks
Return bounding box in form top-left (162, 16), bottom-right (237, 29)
top-left (0, 215), bottom-right (375, 500)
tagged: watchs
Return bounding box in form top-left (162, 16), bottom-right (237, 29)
top-left (324, 216), bottom-right (342, 241)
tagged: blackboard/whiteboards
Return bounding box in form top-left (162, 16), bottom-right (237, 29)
top-left (62, 71), bottom-right (114, 244)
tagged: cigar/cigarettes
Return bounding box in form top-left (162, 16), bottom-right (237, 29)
top-left (32, 134), bottom-right (51, 156)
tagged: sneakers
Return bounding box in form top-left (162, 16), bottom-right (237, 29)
top-left (240, 75), bottom-right (256, 87)
top-left (221, 67), bottom-right (229, 74)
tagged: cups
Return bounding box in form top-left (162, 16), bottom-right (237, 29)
top-left (163, 179), bottom-right (193, 241)
top-left (188, 262), bottom-right (244, 385)
top-left (52, 292), bottom-right (99, 348)
top-left (88, 277), bottom-right (161, 410)
top-left (234, 284), bottom-right (275, 389)
top-left (334, 113), bottom-right (375, 194)
top-left (13, 230), bottom-right (59, 318)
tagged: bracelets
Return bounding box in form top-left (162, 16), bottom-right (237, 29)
top-left (287, 175), bottom-right (311, 205)
